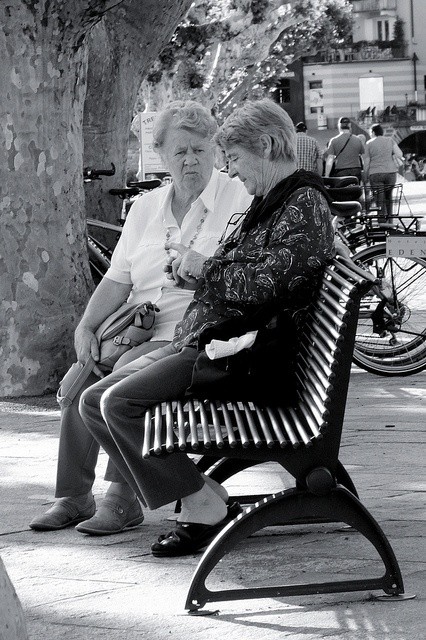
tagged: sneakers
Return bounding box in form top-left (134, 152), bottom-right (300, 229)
top-left (29, 495), bottom-right (96, 531)
top-left (75, 493), bottom-right (144, 535)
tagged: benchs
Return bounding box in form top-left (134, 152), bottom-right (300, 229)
top-left (142, 255), bottom-right (415, 616)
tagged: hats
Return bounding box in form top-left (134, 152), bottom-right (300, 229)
top-left (340, 117), bottom-right (350, 123)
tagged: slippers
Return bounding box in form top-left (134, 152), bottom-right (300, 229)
top-left (151, 501), bottom-right (242, 557)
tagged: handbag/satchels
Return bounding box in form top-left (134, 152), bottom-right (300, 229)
top-left (186, 336), bottom-right (299, 409)
top-left (56, 301), bottom-right (160, 409)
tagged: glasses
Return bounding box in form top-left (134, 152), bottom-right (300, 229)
top-left (219, 206), bottom-right (258, 245)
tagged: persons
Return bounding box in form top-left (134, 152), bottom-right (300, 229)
top-left (295, 122), bottom-right (324, 176)
top-left (324, 117), bottom-right (365, 190)
top-left (28, 100), bottom-right (252, 533)
top-left (78, 99), bottom-right (334, 557)
top-left (362, 124), bottom-right (403, 224)
